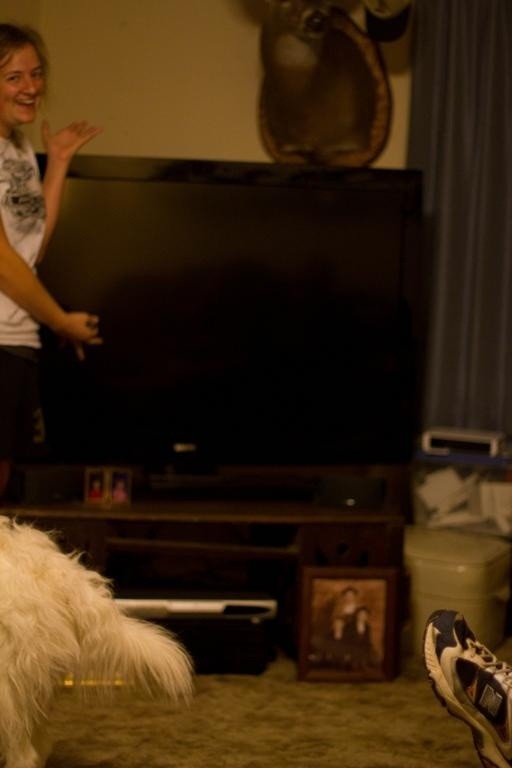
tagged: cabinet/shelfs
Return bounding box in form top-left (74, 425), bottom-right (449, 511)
top-left (2, 458), bottom-right (410, 656)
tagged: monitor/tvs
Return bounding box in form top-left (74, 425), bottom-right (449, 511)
top-left (0, 152), bottom-right (423, 465)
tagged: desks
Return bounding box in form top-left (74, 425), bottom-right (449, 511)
top-left (114, 596), bottom-right (279, 677)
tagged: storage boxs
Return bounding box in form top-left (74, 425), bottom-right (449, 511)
top-left (410, 454), bottom-right (510, 538)
top-left (401, 523), bottom-right (511, 653)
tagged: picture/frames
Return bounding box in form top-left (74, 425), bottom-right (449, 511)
top-left (84, 465), bottom-right (132, 507)
top-left (296, 564), bottom-right (400, 685)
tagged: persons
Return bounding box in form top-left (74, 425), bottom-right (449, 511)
top-left (88, 476), bottom-right (102, 503)
top-left (112, 478), bottom-right (126, 503)
top-left (311, 586), bottom-right (374, 672)
top-left (0, 21), bottom-right (104, 510)
